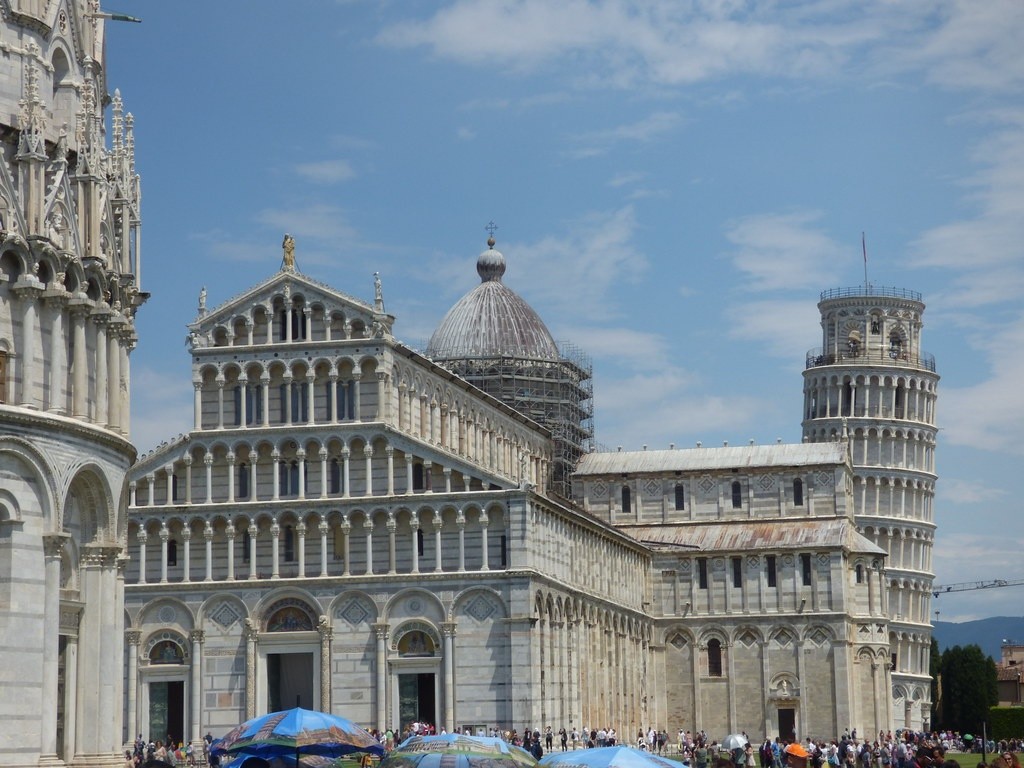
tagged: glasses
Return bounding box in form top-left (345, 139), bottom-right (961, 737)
top-left (926, 756), bottom-right (934, 764)
top-left (1005, 758), bottom-right (1011, 761)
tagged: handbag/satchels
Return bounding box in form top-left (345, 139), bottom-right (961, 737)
top-left (828, 756), bottom-right (835, 765)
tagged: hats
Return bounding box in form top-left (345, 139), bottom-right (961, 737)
top-left (786, 744), bottom-right (808, 757)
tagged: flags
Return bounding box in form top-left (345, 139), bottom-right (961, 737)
top-left (863, 239), bottom-right (866, 262)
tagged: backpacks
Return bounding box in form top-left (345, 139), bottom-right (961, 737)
top-left (571, 733), bottom-right (575, 739)
top-left (534, 744), bottom-right (543, 756)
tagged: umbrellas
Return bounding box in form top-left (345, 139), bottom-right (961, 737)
top-left (964, 734), bottom-right (973, 740)
top-left (381, 733), bottom-right (541, 768)
top-left (787, 742), bottom-right (814, 758)
top-left (537, 744), bottom-right (690, 768)
top-left (209, 707), bottom-right (386, 768)
top-left (722, 733), bottom-right (749, 750)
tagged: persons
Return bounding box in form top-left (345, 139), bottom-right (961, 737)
top-left (113, 301), bottom-right (121, 316)
top-left (477, 727), bottom-right (757, 768)
top-left (283, 285), bottom-right (289, 297)
top-left (372, 272), bottom-right (383, 299)
top-left (100, 289), bottom-right (112, 310)
top-left (54, 271), bottom-right (66, 291)
top-left (759, 735), bottom-right (808, 768)
top-left (48, 213), bottom-right (63, 249)
top-left (361, 720), bottom-right (470, 768)
top-left (78, 280), bottom-right (91, 298)
top-left (25, 263), bottom-right (40, 281)
top-left (282, 233), bottom-right (295, 265)
top-left (806, 728), bottom-right (1024, 768)
top-left (199, 285), bottom-right (207, 307)
top-left (124, 307), bottom-right (135, 327)
top-left (125, 728), bottom-right (212, 768)
